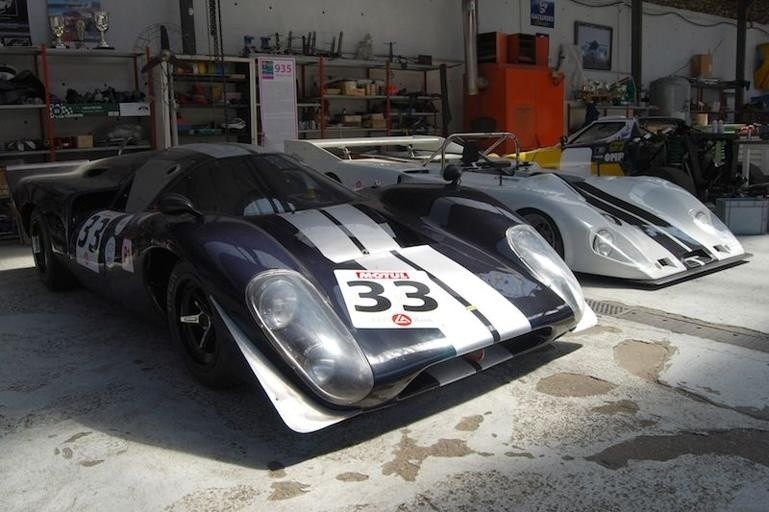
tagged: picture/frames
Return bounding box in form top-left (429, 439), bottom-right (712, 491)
top-left (573, 20), bottom-right (613, 70)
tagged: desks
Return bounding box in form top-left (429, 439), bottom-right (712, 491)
top-left (733, 137), bottom-right (769, 188)
top-left (697, 131), bottom-right (740, 185)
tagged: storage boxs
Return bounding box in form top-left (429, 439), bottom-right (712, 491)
top-left (716, 197), bottom-right (769, 236)
top-left (691, 54), bottom-right (713, 80)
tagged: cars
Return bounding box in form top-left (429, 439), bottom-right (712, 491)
top-left (487, 113), bottom-right (764, 248)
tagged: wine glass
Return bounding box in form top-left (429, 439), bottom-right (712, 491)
top-left (50, 16), bottom-right (67, 50)
top-left (96, 12), bottom-right (110, 48)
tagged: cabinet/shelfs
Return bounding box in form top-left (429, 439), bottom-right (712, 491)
top-left (568, 103), bottom-right (650, 135)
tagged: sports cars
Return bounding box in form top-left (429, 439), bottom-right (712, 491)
top-left (281, 114), bottom-right (753, 295)
top-left (24, 138), bottom-right (596, 444)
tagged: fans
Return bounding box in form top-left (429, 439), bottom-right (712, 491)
top-left (132, 21), bottom-right (198, 147)
